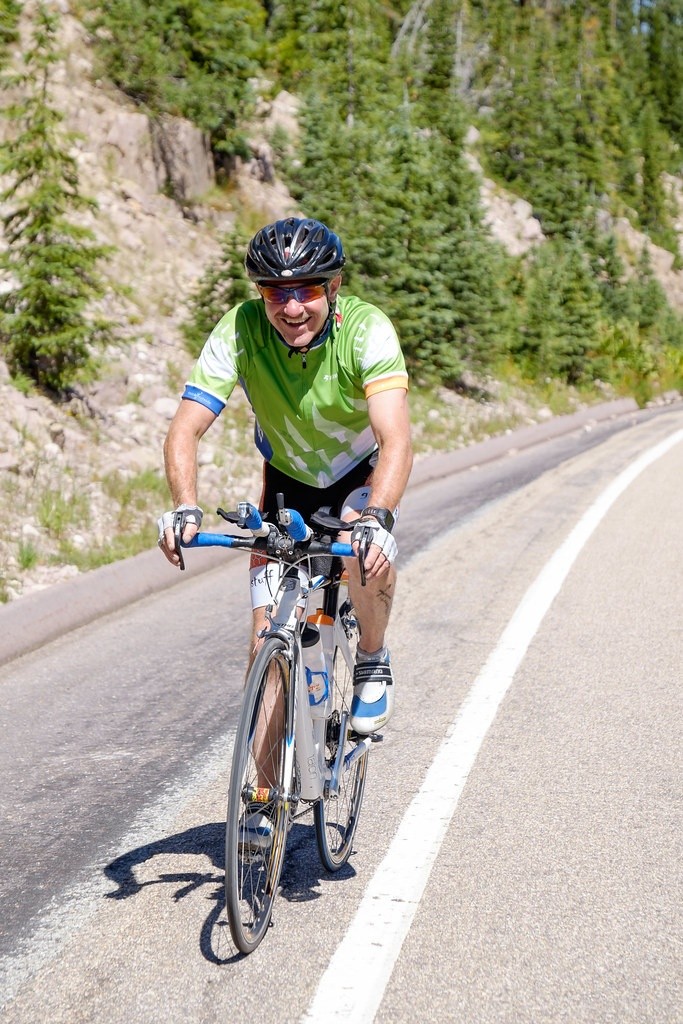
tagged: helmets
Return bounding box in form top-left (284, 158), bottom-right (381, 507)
top-left (245, 218), bottom-right (345, 285)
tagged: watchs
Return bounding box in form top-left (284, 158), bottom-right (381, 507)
top-left (359, 506), bottom-right (394, 532)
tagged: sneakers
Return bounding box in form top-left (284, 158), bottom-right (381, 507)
top-left (349, 641), bottom-right (395, 736)
top-left (235, 802), bottom-right (275, 850)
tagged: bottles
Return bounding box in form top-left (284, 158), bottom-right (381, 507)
top-left (304, 609), bottom-right (333, 719)
top-left (299, 621), bottom-right (331, 706)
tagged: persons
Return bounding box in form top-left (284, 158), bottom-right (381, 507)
top-left (157, 218), bottom-right (413, 851)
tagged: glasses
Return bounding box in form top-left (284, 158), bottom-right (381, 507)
top-left (257, 283), bottom-right (329, 303)
